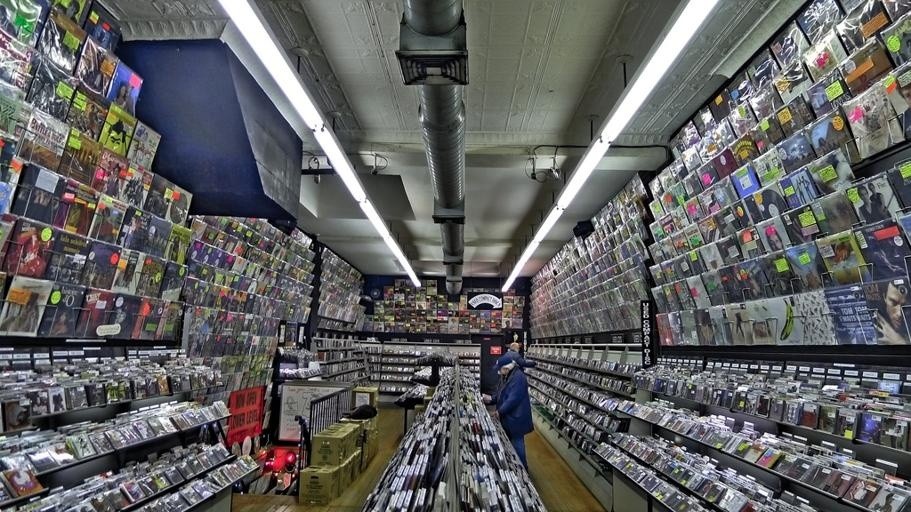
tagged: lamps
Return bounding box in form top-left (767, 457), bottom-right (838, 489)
top-left (348, 152), bottom-right (390, 176)
top-left (522, 146), bottom-right (586, 184)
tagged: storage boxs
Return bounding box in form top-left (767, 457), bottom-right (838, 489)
top-left (299, 386), bottom-right (377, 507)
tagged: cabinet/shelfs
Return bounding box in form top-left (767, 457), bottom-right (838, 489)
top-left (524, 344), bottom-right (896, 512)
top-left (2, 339), bottom-right (259, 512)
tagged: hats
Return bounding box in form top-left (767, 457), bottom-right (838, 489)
top-left (492, 356), bottom-right (512, 371)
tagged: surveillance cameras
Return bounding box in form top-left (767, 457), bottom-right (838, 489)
top-left (314, 175), bottom-right (320, 184)
top-left (549, 168), bottom-right (561, 181)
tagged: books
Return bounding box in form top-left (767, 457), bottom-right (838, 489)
top-left (1, 0), bottom-right (911, 512)
